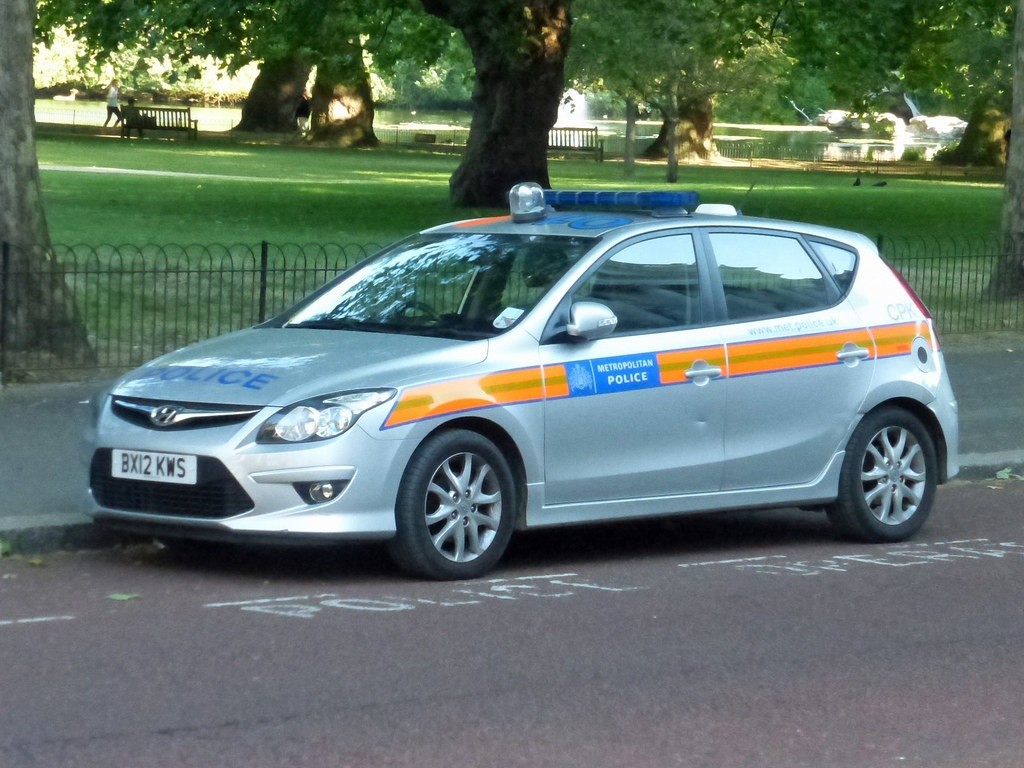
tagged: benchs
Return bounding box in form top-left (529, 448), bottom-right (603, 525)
top-left (549, 126), bottom-right (605, 162)
top-left (120, 103), bottom-right (199, 142)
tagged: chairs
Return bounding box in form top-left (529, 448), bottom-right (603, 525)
top-left (513, 243), bottom-right (569, 309)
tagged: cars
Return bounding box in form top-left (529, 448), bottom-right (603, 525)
top-left (83, 180), bottom-right (962, 579)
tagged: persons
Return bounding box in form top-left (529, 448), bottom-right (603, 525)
top-left (295, 87), bottom-right (312, 132)
top-left (104, 79), bottom-right (146, 139)
